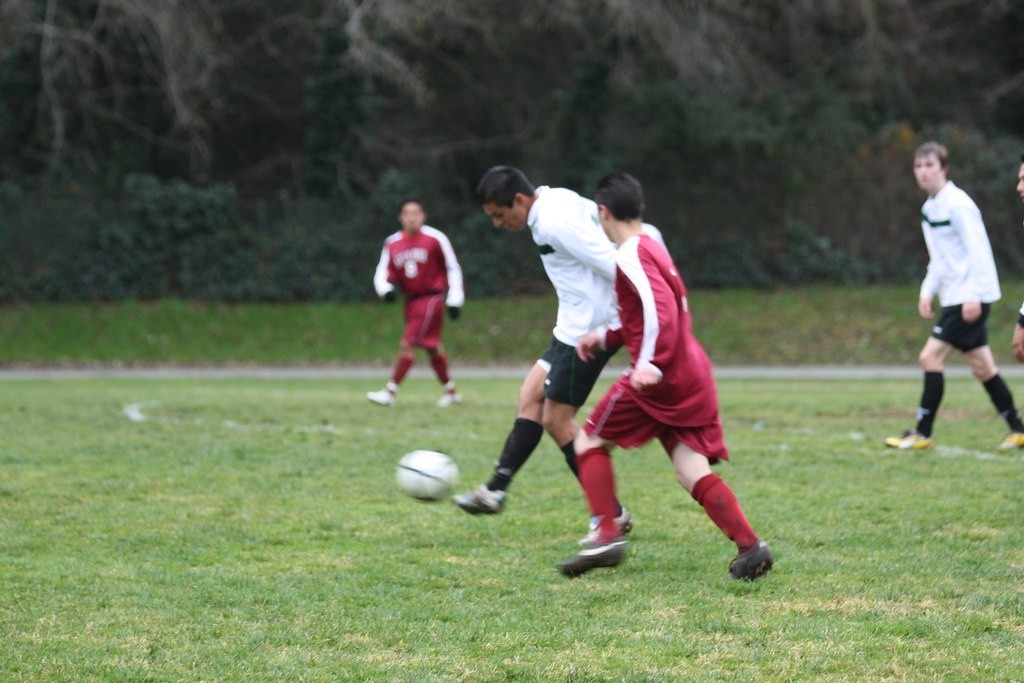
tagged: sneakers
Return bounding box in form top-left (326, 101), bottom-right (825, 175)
top-left (438, 391), bottom-right (461, 406)
top-left (558, 538), bottom-right (627, 576)
top-left (450, 487), bottom-right (505, 516)
top-left (997, 433), bottom-right (1024, 451)
top-left (730, 541), bottom-right (774, 580)
top-left (885, 430), bottom-right (933, 448)
top-left (578, 507), bottom-right (633, 546)
top-left (366, 390), bottom-right (396, 407)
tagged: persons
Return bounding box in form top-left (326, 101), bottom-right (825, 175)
top-left (885, 142), bottom-right (1024, 452)
top-left (556, 172), bottom-right (773, 582)
top-left (451, 165), bottom-right (661, 535)
top-left (1012, 153), bottom-right (1024, 364)
top-left (365, 197), bottom-right (465, 407)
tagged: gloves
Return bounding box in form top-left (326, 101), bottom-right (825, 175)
top-left (386, 292), bottom-right (397, 302)
top-left (448, 307), bottom-right (460, 320)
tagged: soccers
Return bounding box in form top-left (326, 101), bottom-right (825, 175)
top-left (396, 449), bottom-right (461, 501)
